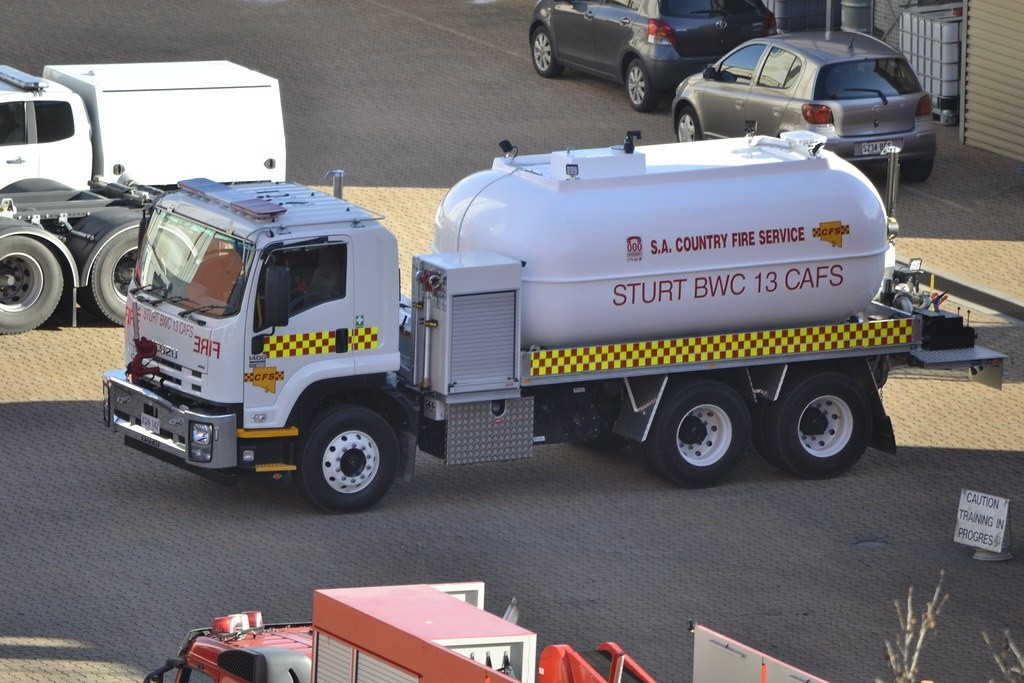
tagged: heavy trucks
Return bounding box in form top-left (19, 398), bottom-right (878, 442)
top-left (98, 129), bottom-right (1023, 517)
top-left (1, 62), bottom-right (287, 334)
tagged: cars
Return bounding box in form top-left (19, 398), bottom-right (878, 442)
top-left (529, 0), bottom-right (777, 114)
top-left (670, 32), bottom-right (937, 183)
